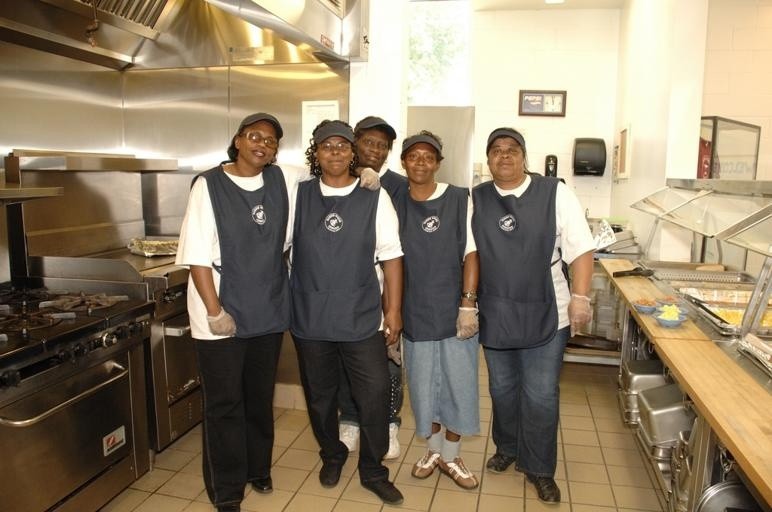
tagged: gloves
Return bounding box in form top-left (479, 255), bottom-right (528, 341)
top-left (385, 341), bottom-right (403, 365)
top-left (456, 307), bottom-right (482, 339)
top-left (206, 306), bottom-right (237, 338)
top-left (568, 294), bottom-right (593, 338)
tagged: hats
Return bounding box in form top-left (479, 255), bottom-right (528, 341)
top-left (487, 129), bottom-right (526, 154)
top-left (237, 112), bottom-right (286, 136)
top-left (359, 117), bottom-right (398, 137)
top-left (401, 136), bottom-right (443, 155)
top-left (313, 122), bottom-right (355, 144)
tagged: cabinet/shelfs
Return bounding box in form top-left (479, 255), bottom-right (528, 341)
top-left (689, 114), bottom-right (764, 273)
top-left (598, 175), bottom-right (772, 511)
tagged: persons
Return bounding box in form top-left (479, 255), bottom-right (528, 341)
top-left (356, 129), bottom-right (481, 490)
top-left (174, 112), bottom-right (295, 512)
top-left (338, 116), bottom-right (404, 460)
top-left (281, 120), bottom-right (407, 504)
top-left (472, 126), bottom-right (596, 504)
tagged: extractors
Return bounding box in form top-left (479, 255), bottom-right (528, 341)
top-left (1, 1), bottom-right (183, 73)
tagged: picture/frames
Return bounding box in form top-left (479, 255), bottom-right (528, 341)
top-left (614, 123), bottom-right (633, 180)
top-left (515, 88), bottom-right (568, 119)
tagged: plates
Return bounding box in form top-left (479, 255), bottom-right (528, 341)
top-left (630, 295), bottom-right (689, 328)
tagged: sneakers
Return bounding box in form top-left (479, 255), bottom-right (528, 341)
top-left (382, 422), bottom-right (403, 460)
top-left (525, 469), bottom-right (564, 505)
top-left (487, 448), bottom-right (516, 475)
top-left (358, 477), bottom-right (405, 507)
top-left (337, 420), bottom-right (359, 454)
top-left (316, 460), bottom-right (343, 489)
top-left (411, 448), bottom-right (480, 489)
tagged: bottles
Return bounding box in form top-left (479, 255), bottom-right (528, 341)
top-left (612, 321), bottom-right (623, 351)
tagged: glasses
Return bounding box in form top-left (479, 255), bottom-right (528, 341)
top-left (320, 141), bottom-right (350, 152)
top-left (241, 130), bottom-right (280, 151)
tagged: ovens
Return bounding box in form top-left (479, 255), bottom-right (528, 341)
top-left (151, 289), bottom-right (203, 454)
top-left (0, 340), bottom-right (152, 512)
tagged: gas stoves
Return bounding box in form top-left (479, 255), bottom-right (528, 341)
top-left (0, 282), bottom-right (160, 396)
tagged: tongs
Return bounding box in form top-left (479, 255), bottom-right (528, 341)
top-left (613, 266), bottom-right (655, 278)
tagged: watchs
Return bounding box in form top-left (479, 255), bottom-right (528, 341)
top-left (461, 291), bottom-right (478, 302)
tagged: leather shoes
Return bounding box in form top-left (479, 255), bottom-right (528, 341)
top-left (218, 506), bottom-right (239, 512)
top-left (250, 476), bottom-right (275, 494)
